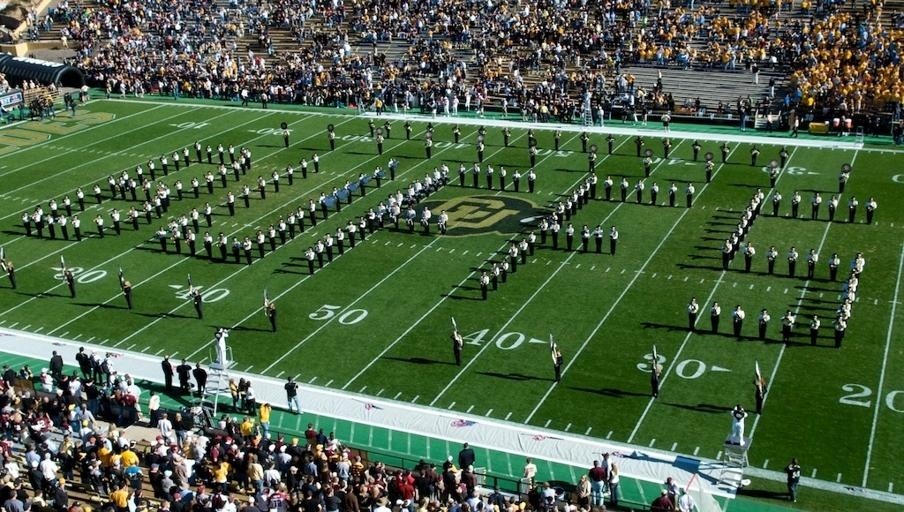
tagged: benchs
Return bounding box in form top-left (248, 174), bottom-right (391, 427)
top-left (23, 86), bottom-right (64, 107)
top-left (19, 0), bottom-right (903, 116)
top-left (0, 466), bottom-right (255, 512)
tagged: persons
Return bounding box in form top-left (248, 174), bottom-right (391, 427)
top-left (731, 404), bottom-right (748, 446)
top-left (451, 332), bottom-right (464, 365)
top-left (20, 118), bottom-right (878, 348)
top-left (1, 2), bottom-right (904, 121)
top-left (192, 289), bottom-right (203, 319)
top-left (123, 280), bottom-right (132, 309)
top-left (656, 477), bottom-right (694, 511)
top-left (753, 374), bottom-right (767, 413)
top-left (786, 459), bottom-right (801, 502)
top-left (1, 345), bottom-right (621, 510)
top-left (554, 349), bottom-right (563, 381)
top-left (268, 303), bottom-right (277, 333)
top-left (65, 271), bottom-right (76, 298)
top-left (652, 364), bottom-right (659, 398)
top-left (214, 326), bottom-right (229, 365)
top-left (4, 258), bottom-right (16, 289)
